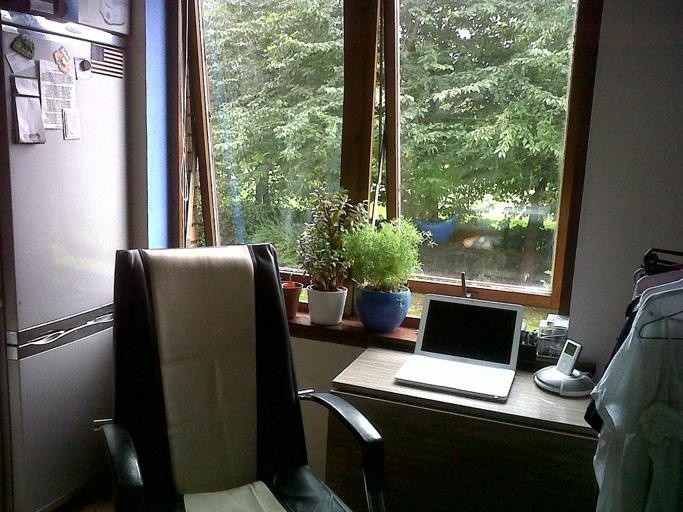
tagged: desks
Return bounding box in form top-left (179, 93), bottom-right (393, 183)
top-left (325, 345), bottom-right (601, 510)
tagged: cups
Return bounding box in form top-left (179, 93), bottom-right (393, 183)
top-left (282, 282), bottom-right (305, 320)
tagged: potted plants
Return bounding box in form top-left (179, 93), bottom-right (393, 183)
top-left (340, 221), bottom-right (436, 329)
top-left (296, 188), bottom-right (380, 324)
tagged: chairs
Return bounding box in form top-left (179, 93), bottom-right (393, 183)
top-left (93, 242), bottom-right (387, 509)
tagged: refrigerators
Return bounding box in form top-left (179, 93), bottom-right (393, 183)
top-left (0, 6), bottom-right (130, 511)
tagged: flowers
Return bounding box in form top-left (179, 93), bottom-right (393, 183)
top-left (285, 282), bottom-right (295, 287)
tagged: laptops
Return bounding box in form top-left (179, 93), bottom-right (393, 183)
top-left (395, 292), bottom-right (523, 402)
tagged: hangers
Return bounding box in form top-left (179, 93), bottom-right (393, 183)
top-left (622, 245), bottom-right (682, 342)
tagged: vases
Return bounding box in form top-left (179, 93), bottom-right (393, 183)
top-left (279, 281), bottom-right (304, 318)
